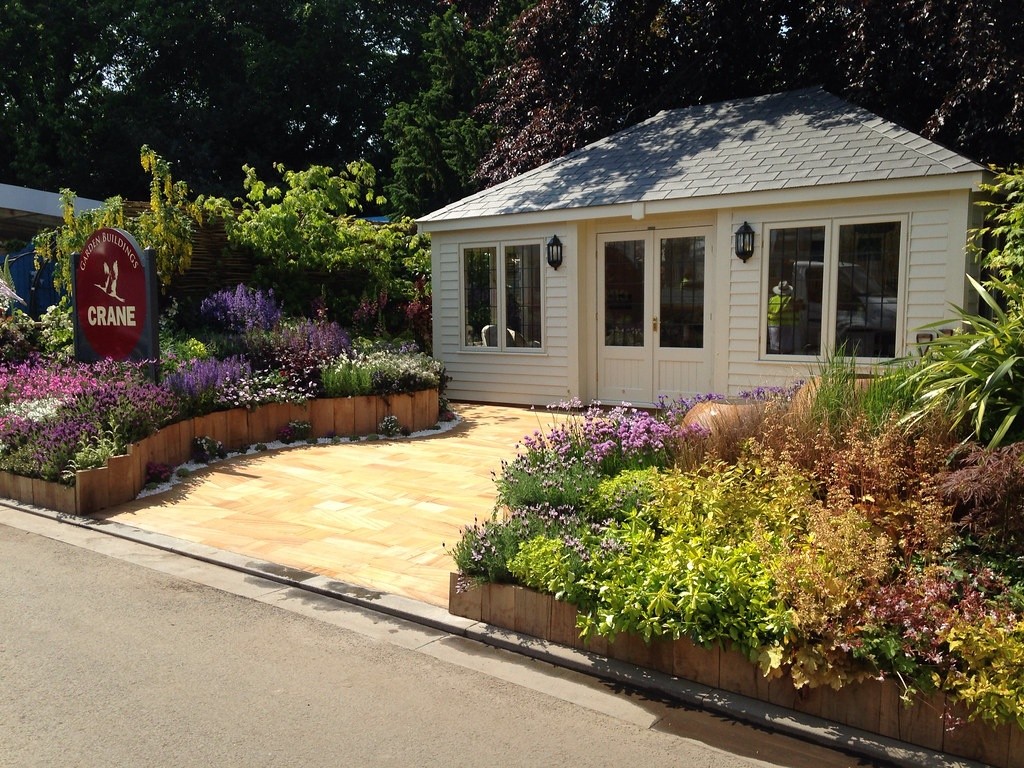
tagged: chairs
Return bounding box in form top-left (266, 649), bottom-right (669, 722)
top-left (480, 324), bottom-right (541, 347)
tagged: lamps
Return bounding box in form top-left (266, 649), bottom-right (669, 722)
top-left (547, 234), bottom-right (563, 270)
top-left (734, 221), bottom-right (755, 263)
